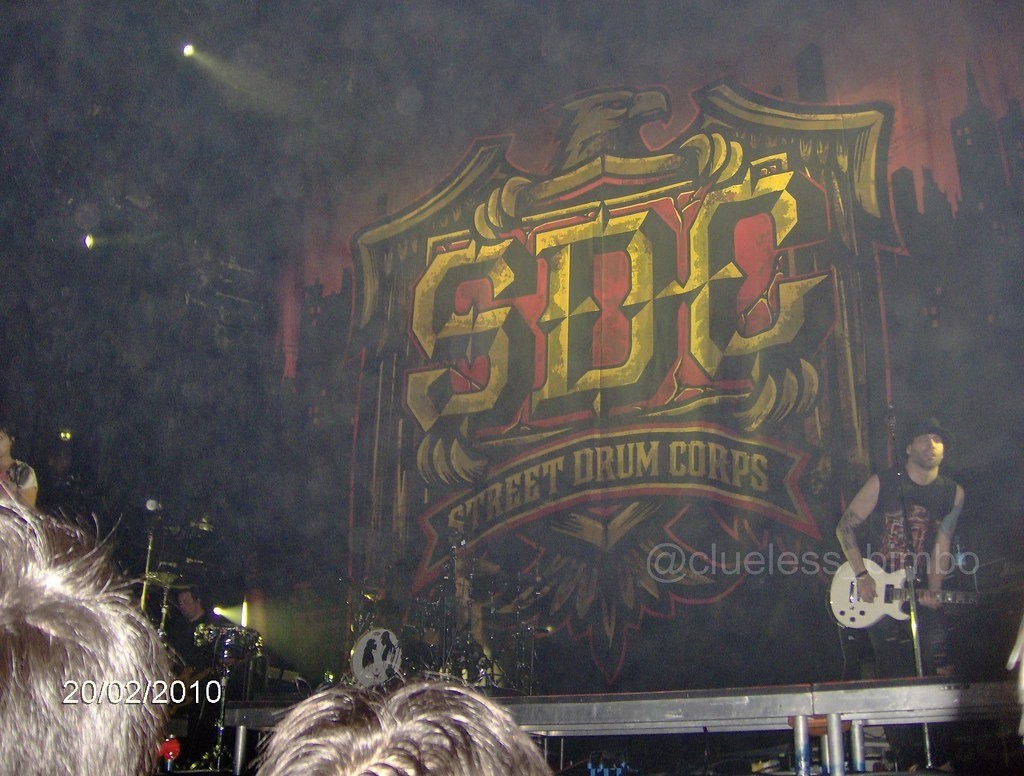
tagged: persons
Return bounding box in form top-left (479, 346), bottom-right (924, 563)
top-left (255, 680), bottom-right (553, 776)
top-left (0, 419), bottom-right (38, 507)
top-left (0, 500), bottom-right (173, 776)
top-left (836, 418), bottom-right (964, 771)
top-left (172, 587), bottom-right (240, 769)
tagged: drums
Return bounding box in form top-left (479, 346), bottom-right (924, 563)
top-left (133, 500), bottom-right (219, 593)
top-left (347, 628), bottom-right (405, 688)
top-left (192, 622), bottom-right (265, 667)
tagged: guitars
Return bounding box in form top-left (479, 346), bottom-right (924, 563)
top-left (823, 556), bottom-right (988, 631)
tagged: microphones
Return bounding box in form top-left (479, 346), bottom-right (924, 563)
top-left (884, 406), bottom-right (896, 424)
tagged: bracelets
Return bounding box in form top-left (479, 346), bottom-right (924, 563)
top-left (856, 570), bottom-right (867, 578)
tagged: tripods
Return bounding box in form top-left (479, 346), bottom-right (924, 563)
top-left (190, 668), bottom-right (235, 772)
top-left (441, 528), bottom-right (547, 697)
